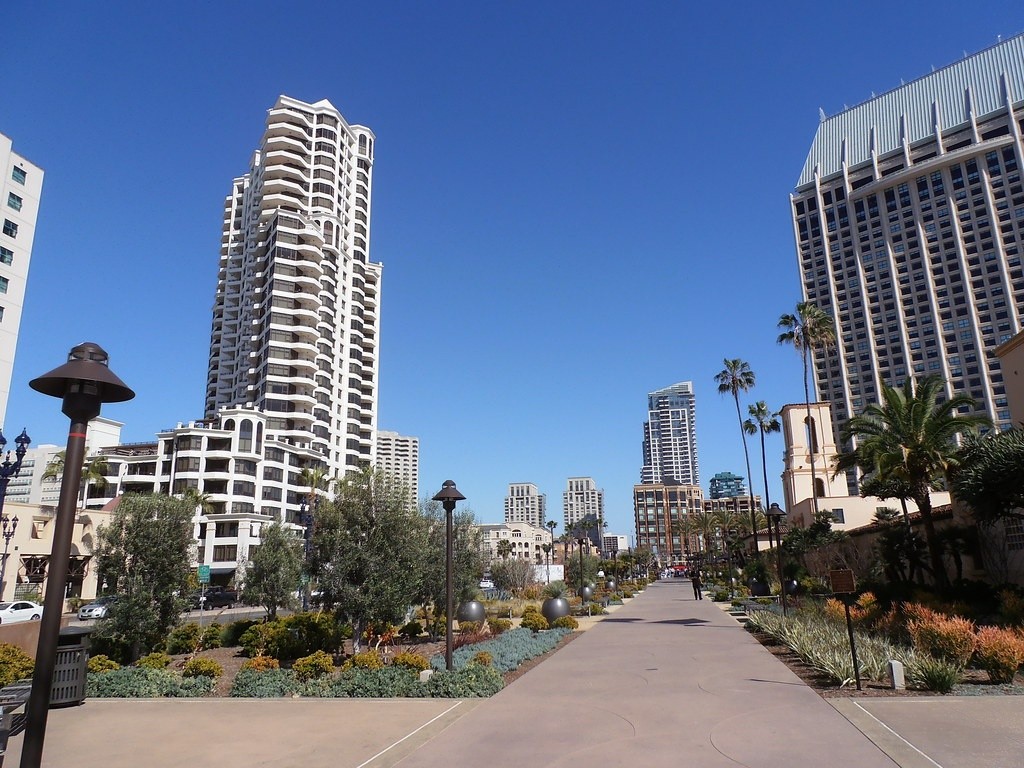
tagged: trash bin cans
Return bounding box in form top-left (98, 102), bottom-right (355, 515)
top-left (49, 626), bottom-right (94, 708)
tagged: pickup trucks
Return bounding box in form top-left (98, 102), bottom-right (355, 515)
top-left (171, 586), bottom-right (238, 611)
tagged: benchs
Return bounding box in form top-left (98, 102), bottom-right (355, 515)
top-left (0, 679), bottom-right (33, 707)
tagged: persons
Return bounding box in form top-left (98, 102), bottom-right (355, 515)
top-left (692, 567), bottom-right (703, 600)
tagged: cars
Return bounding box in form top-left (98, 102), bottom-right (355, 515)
top-left (0, 600), bottom-right (44, 625)
top-left (479, 580), bottom-right (495, 588)
top-left (77, 596), bottom-right (118, 621)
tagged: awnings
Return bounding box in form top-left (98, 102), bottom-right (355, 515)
top-left (70, 543), bottom-right (92, 556)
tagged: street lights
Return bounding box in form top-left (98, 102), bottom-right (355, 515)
top-left (574, 532), bottom-right (588, 606)
top-left (612, 548), bottom-right (620, 592)
top-left (19, 342), bottom-right (135, 768)
top-left (765, 503), bottom-right (788, 617)
top-left (431, 480), bottom-right (467, 673)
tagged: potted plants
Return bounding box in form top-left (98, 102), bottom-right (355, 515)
top-left (721, 560), bottom-right (809, 596)
top-left (456, 575), bottom-right (616, 633)
